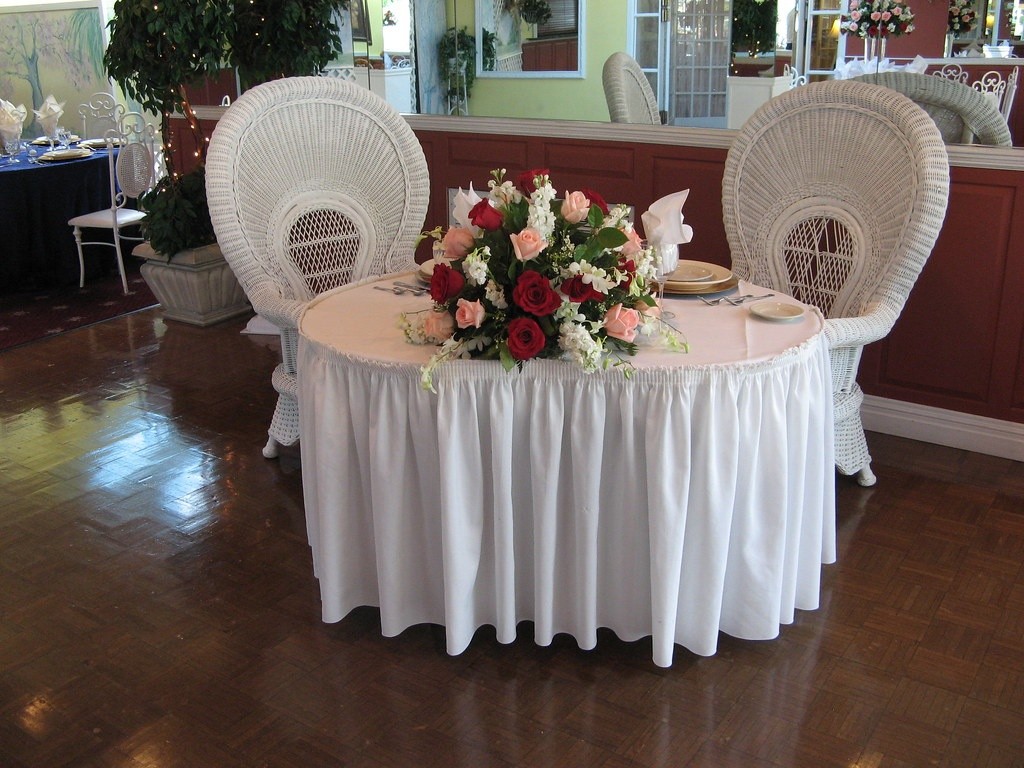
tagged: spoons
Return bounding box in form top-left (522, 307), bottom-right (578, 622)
top-left (395, 285), bottom-right (426, 296)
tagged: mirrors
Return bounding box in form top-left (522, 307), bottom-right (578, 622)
top-left (475, 0), bottom-right (585, 79)
top-left (944, 0), bottom-right (1024, 59)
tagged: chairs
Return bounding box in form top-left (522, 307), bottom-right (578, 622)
top-left (848, 72), bottom-right (1014, 147)
top-left (67, 112), bottom-right (164, 294)
top-left (76, 91), bottom-right (125, 140)
top-left (602, 51), bottom-right (662, 126)
top-left (721, 80), bottom-right (951, 489)
top-left (204, 76), bottom-right (430, 460)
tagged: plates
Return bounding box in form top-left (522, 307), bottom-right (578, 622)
top-left (39, 151), bottom-right (94, 160)
top-left (80, 137), bottom-right (124, 147)
top-left (750, 301), bottom-right (806, 321)
top-left (668, 264), bottom-right (714, 282)
top-left (32, 137), bottom-right (81, 144)
top-left (650, 259), bottom-right (733, 290)
top-left (650, 273), bottom-right (738, 295)
top-left (415, 259), bottom-right (435, 284)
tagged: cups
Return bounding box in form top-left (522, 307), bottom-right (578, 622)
top-left (26, 143), bottom-right (38, 164)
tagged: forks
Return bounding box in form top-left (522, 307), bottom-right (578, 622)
top-left (373, 286), bottom-right (409, 294)
top-left (723, 294), bottom-right (775, 306)
top-left (27, 157), bottom-right (48, 165)
top-left (696, 294), bottom-right (754, 306)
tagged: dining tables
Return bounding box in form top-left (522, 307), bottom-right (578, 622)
top-left (0, 137), bottom-right (136, 294)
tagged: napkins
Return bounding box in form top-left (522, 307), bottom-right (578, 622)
top-left (32, 134), bottom-right (81, 145)
top-left (39, 149), bottom-right (90, 161)
top-left (32, 95), bottom-right (68, 137)
top-left (77, 137), bottom-right (125, 148)
top-left (0, 97), bottom-right (28, 144)
top-left (638, 189), bottom-right (693, 274)
top-left (451, 182), bottom-right (489, 239)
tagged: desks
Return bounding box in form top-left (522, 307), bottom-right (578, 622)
top-left (296, 258), bottom-right (837, 668)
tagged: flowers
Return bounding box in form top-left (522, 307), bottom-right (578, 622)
top-left (946, 0), bottom-right (981, 40)
top-left (839, 0), bottom-right (916, 39)
top-left (373, 167), bottom-right (692, 394)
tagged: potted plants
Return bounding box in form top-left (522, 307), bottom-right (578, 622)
top-left (436, 26), bottom-right (476, 100)
top-left (102, 0), bottom-right (254, 326)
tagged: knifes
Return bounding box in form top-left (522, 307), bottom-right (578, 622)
top-left (393, 281), bottom-right (431, 293)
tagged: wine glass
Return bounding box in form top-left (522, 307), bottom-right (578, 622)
top-left (5, 139), bottom-right (20, 164)
top-left (58, 132), bottom-right (72, 150)
